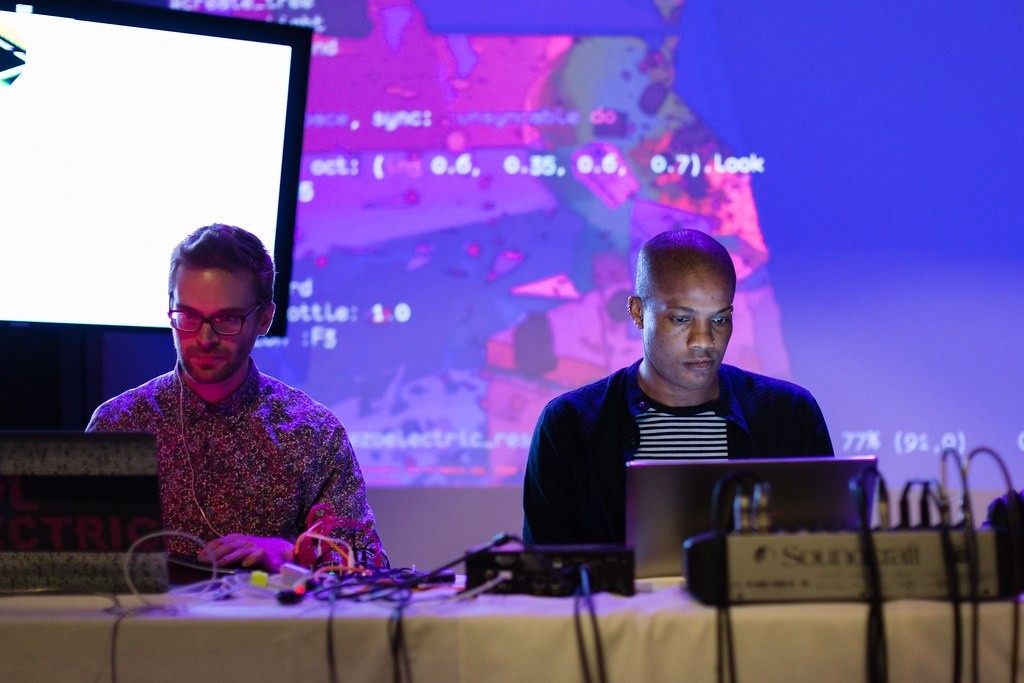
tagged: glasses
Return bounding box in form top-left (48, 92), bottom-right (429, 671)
top-left (168, 292), bottom-right (265, 335)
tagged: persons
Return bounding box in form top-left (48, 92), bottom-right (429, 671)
top-left (522, 228), bottom-right (835, 544)
top-left (84, 223), bottom-right (390, 570)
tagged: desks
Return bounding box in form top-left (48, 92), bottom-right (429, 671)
top-left (0, 565), bottom-right (1024, 683)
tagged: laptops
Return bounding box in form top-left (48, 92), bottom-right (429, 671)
top-left (0, 431), bottom-right (268, 596)
top-left (624, 455), bottom-right (876, 579)
